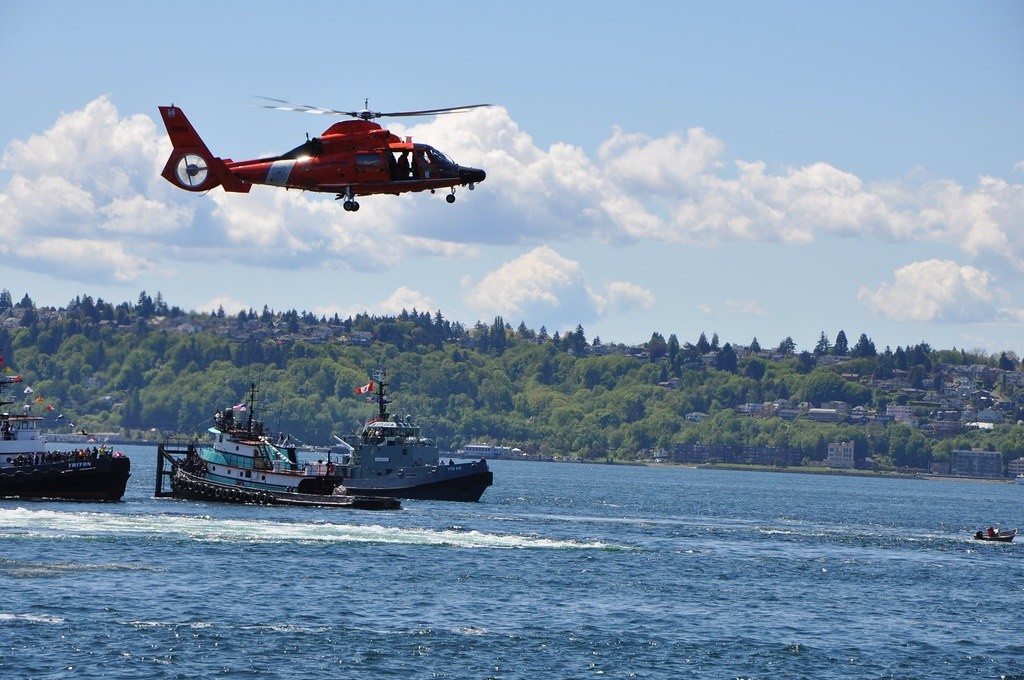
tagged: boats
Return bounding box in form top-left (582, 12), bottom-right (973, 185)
top-left (0, 347), bottom-right (131, 503)
top-left (456, 442), bottom-right (501, 460)
top-left (307, 364), bottom-right (494, 502)
top-left (1007, 474), bottom-right (1024, 486)
top-left (169, 360), bottom-right (402, 510)
top-left (974, 526), bottom-right (1018, 543)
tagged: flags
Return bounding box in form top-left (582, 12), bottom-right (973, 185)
top-left (24, 387), bottom-right (95, 442)
top-left (233, 402), bottom-right (248, 411)
top-left (0, 357), bottom-right (22, 383)
top-left (367, 396), bottom-right (375, 402)
top-left (356, 381), bottom-right (374, 393)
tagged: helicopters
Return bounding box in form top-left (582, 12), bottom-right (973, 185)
top-left (156, 93), bottom-right (493, 212)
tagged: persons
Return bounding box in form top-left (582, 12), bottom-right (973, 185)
top-left (386, 149), bottom-right (396, 177)
top-left (362, 427), bottom-right (382, 436)
top-left (418, 151), bottom-right (442, 178)
top-left (17, 444), bottom-right (113, 466)
top-left (214, 409), bottom-right (233, 422)
top-left (399, 151), bottom-right (412, 179)
top-left (1, 424), bottom-right (16, 439)
top-left (975, 531), bottom-right (983, 540)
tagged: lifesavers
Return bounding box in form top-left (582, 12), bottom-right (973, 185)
top-left (171, 475), bottom-right (276, 506)
top-left (0, 469), bottom-right (59, 484)
